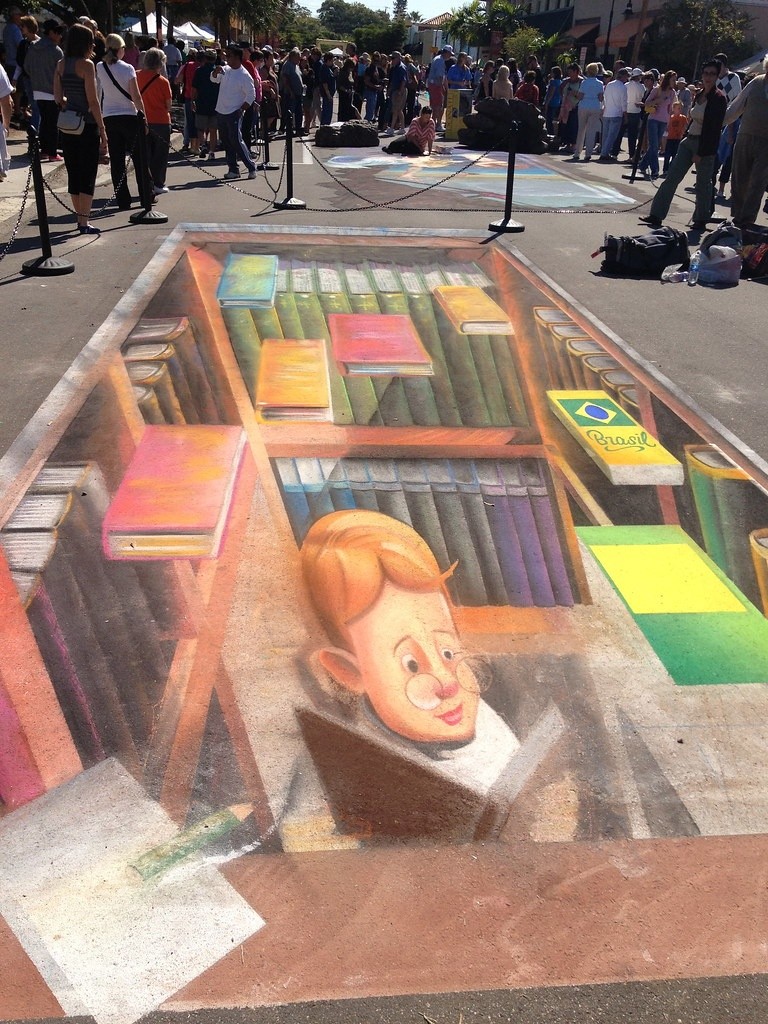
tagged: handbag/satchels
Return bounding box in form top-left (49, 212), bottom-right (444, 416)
top-left (589, 226), bottom-right (691, 278)
top-left (737, 228), bottom-right (768, 284)
top-left (56, 108), bottom-right (86, 135)
top-left (644, 87), bottom-right (662, 114)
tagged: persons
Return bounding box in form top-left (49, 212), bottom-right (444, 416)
top-left (382, 106), bottom-right (437, 156)
top-left (683, 54), bottom-right (768, 232)
top-left (1, 8), bottom-right (187, 233)
top-left (597, 67), bottom-right (704, 177)
top-left (639, 60), bottom-right (726, 229)
top-left (426, 45), bottom-right (629, 162)
top-left (175, 41), bottom-right (430, 178)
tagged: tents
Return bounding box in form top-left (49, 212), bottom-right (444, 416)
top-left (121, 13), bottom-right (220, 45)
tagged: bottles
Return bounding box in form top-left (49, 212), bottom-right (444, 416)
top-left (668, 271), bottom-right (689, 282)
top-left (689, 254), bottom-right (700, 283)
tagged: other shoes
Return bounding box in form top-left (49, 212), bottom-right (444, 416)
top-left (685, 222), bottom-right (706, 230)
top-left (140, 196), bottom-right (159, 206)
top-left (77, 222), bottom-right (101, 234)
top-left (153, 185), bottom-right (169, 194)
top-left (0, 170), bottom-right (8, 183)
top-left (558, 143), bottom-right (726, 198)
top-left (119, 204), bottom-right (131, 210)
top-left (639, 214), bottom-right (662, 226)
top-left (181, 122), bottom-right (446, 161)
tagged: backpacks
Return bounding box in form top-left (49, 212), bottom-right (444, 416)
top-left (696, 219), bottom-right (743, 256)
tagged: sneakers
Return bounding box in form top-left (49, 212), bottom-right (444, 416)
top-left (247, 170), bottom-right (258, 179)
top-left (41, 153), bottom-right (50, 160)
top-left (224, 171), bottom-right (242, 179)
top-left (49, 154), bottom-right (64, 161)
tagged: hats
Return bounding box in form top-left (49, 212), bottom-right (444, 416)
top-left (389, 51), bottom-right (401, 57)
top-left (650, 68), bottom-right (661, 76)
top-left (442, 44), bottom-right (455, 56)
top-left (41, 19), bottom-right (68, 32)
top-left (675, 76), bottom-right (687, 85)
top-left (687, 84), bottom-right (698, 91)
top-left (625, 66), bottom-right (632, 74)
top-left (602, 69), bottom-right (613, 78)
top-left (630, 68), bottom-right (645, 77)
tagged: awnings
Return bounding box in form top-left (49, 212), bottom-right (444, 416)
top-left (560, 22), bottom-right (599, 48)
top-left (595, 18), bottom-right (654, 47)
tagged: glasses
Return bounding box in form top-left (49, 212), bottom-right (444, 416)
top-left (701, 72), bottom-right (718, 78)
